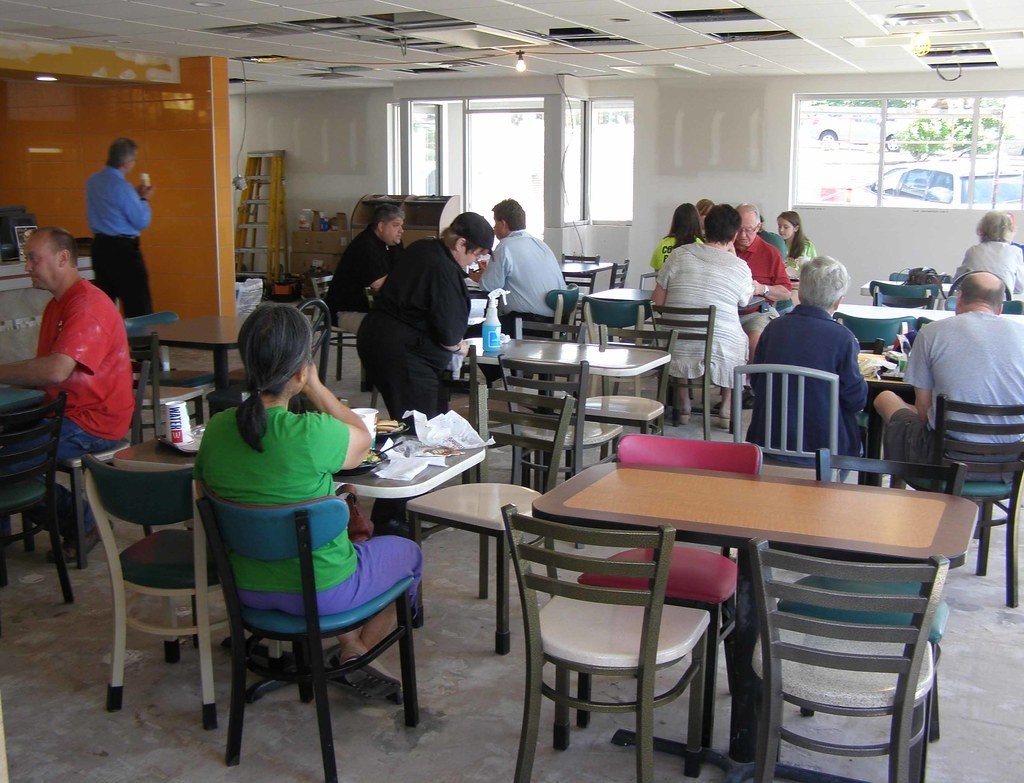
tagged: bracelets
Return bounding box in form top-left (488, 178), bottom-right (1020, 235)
top-left (141, 197), bottom-right (148, 201)
top-left (452, 346), bottom-right (461, 354)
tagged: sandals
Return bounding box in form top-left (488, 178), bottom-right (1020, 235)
top-left (324, 644), bottom-right (401, 699)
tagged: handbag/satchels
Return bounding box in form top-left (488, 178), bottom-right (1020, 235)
top-left (897, 267), bottom-right (945, 286)
top-left (331, 484), bottom-right (375, 544)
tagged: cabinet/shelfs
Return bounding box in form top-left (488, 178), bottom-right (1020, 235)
top-left (353, 192), bottom-right (460, 247)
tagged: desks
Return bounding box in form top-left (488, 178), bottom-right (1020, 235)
top-left (556, 261), bottom-right (613, 274)
top-left (587, 286), bottom-right (767, 430)
top-left (460, 337), bottom-right (671, 495)
top-left (861, 280), bottom-right (1024, 303)
top-left (831, 304), bottom-right (1024, 323)
top-left (531, 461), bottom-right (980, 783)
top-left (858, 354), bottom-right (916, 488)
top-left (0, 387), bottom-right (47, 412)
top-left (127, 316), bottom-right (325, 422)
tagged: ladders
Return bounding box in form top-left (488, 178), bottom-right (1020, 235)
top-left (236, 150), bottom-right (288, 288)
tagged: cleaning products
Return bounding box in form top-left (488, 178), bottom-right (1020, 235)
top-left (482, 287), bottom-right (511, 353)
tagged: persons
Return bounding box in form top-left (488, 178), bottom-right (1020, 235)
top-left (324, 199), bottom-right (569, 539)
top-left (874, 273), bottom-right (1024, 484)
top-left (745, 255), bottom-right (868, 483)
top-left (85, 136), bottom-right (177, 372)
top-left (192, 303), bottom-right (424, 701)
top-left (954, 212), bottom-right (1024, 302)
top-left (650, 199), bottom-right (814, 429)
top-left (0, 226), bottom-right (135, 562)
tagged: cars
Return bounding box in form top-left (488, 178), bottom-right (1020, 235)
top-left (808, 109), bottom-right (906, 152)
top-left (845, 160), bottom-right (1023, 205)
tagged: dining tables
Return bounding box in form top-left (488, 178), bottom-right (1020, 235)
top-left (112, 430), bottom-right (486, 704)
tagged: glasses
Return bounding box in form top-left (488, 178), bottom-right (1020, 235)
top-left (739, 223), bottom-right (761, 233)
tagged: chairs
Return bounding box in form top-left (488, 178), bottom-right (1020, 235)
top-left (56, 360), bottom-right (153, 569)
top-left (499, 504), bottom-right (710, 783)
top-left (0, 391), bottom-right (74, 605)
top-left (750, 535), bottom-right (950, 783)
top-left (196, 479), bottom-right (419, 783)
top-left (302, 255), bottom-right (1024, 609)
top-left (776, 446), bottom-right (968, 743)
top-left (577, 433), bottom-right (763, 750)
top-left (81, 453), bottom-right (284, 731)
top-left (124, 312), bottom-right (215, 389)
top-left (126, 331), bottom-right (204, 443)
top-left (406, 384), bottom-right (575, 656)
top-left (207, 299), bottom-right (331, 419)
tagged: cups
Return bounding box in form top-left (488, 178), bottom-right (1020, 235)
top-left (351, 408), bottom-right (379, 451)
top-left (165, 401), bottom-right (195, 447)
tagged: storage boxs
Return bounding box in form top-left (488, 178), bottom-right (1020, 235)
top-left (289, 229), bottom-right (349, 279)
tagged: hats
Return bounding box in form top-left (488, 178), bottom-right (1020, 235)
top-left (450, 212), bottom-right (495, 262)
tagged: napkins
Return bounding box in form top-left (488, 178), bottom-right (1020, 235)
top-left (380, 437), bottom-right (446, 467)
top-left (373, 457), bottom-right (428, 482)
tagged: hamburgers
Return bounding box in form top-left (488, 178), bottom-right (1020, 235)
top-left (377, 420), bottom-right (399, 433)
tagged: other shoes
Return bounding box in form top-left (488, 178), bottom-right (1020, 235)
top-left (47, 519), bottom-right (114, 563)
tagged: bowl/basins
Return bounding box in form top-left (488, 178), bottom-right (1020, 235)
top-left (334, 449), bottom-right (387, 477)
top-left (375, 418), bottom-right (411, 441)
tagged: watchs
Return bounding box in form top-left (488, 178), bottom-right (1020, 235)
top-left (762, 285), bottom-right (769, 298)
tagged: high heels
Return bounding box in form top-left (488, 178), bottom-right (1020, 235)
top-left (681, 405), bottom-right (692, 424)
top-left (719, 407), bottom-right (731, 428)
